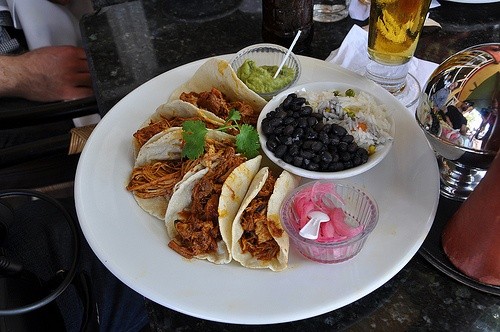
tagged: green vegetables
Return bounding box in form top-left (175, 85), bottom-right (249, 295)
top-left (182, 109), bottom-right (260, 161)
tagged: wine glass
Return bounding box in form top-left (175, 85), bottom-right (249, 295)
top-left (356, 0), bottom-right (432, 107)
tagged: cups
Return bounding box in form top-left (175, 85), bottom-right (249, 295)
top-left (260, 0), bottom-right (371, 54)
top-left (0, 190), bottom-right (80, 314)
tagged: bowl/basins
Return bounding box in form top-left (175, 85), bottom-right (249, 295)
top-left (278, 178), bottom-right (378, 264)
top-left (229, 43), bottom-right (301, 101)
top-left (257, 81), bottom-right (397, 180)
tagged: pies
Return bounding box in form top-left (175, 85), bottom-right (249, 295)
top-left (168, 57), bottom-right (269, 122)
top-left (134, 98), bottom-right (228, 159)
top-left (130, 129), bottom-right (302, 270)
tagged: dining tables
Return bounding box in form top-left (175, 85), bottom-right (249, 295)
top-left (79, 0), bottom-right (500, 332)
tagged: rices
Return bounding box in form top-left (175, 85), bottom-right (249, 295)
top-left (317, 92), bottom-right (387, 153)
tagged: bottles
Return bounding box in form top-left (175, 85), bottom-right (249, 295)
top-left (443, 146), bottom-right (500, 285)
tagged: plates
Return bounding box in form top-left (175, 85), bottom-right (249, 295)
top-left (73, 54), bottom-right (443, 325)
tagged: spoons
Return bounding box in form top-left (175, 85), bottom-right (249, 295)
top-left (298, 210), bottom-right (331, 240)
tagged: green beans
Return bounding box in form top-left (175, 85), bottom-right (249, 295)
top-left (325, 89), bottom-right (356, 119)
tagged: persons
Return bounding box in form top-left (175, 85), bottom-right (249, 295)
top-left (0, 0), bottom-right (93, 110)
top-left (421, 78), bottom-right (500, 153)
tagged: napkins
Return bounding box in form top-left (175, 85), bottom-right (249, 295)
top-left (327, 24), bottom-right (439, 116)
